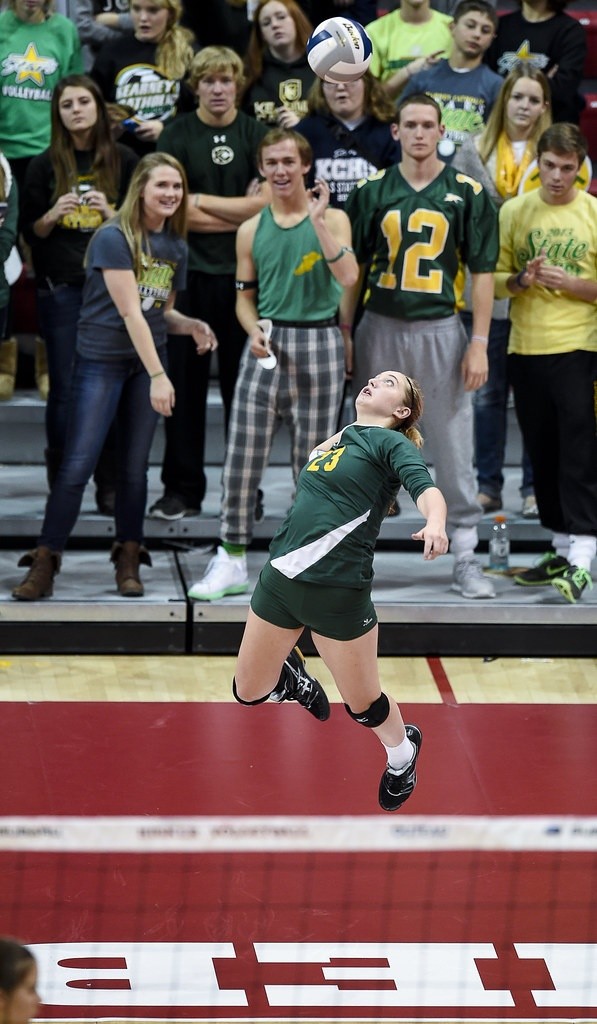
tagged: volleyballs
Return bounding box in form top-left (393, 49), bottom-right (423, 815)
top-left (307, 17), bottom-right (374, 84)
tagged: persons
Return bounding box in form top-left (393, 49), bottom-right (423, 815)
top-left (232, 370), bottom-right (448, 812)
top-left (0, 152), bottom-right (22, 309)
top-left (0, 937), bottom-right (42, 1024)
top-left (15, 76), bottom-right (140, 512)
top-left (0, 0), bottom-right (86, 401)
top-left (495, 123), bottom-right (597, 603)
top-left (12, 154), bottom-right (219, 599)
top-left (49, 0), bottom-right (587, 154)
top-left (188, 128), bottom-right (360, 599)
top-left (149, 46), bottom-right (270, 522)
top-left (340, 93), bottom-right (500, 600)
top-left (285, 65), bottom-right (403, 517)
top-left (450, 63), bottom-right (552, 520)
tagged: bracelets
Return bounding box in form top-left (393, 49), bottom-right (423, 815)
top-left (148, 370), bottom-right (165, 378)
top-left (517, 270), bottom-right (530, 289)
top-left (193, 193), bottom-right (199, 208)
top-left (324, 247), bottom-right (345, 263)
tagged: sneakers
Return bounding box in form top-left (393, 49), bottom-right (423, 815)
top-left (378, 723), bottom-right (422, 812)
top-left (511, 554), bottom-right (570, 586)
top-left (452, 554), bottom-right (496, 598)
top-left (269, 645), bottom-right (331, 721)
top-left (552, 561), bottom-right (592, 605)
top-left (188, 547), bottom-right (250, 601)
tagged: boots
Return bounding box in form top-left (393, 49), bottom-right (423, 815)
top-left (107, 540), bottom-right (153, 597)
top-left (12, 545), bottom-right (61, 600)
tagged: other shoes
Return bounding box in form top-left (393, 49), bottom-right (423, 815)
top-left (475, 491), bottom-right (503, 515)
top-left (523, 494), bottom-right (538, 517)
top-left (149, 497), bottom-right (201, 522)
top-left (252, 488), bottom-right (267, 523)
top-left (95, 490), bottom-right (115, 514)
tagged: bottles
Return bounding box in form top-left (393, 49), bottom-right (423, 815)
top-left (488, 513), bottom-right (510, 572)
